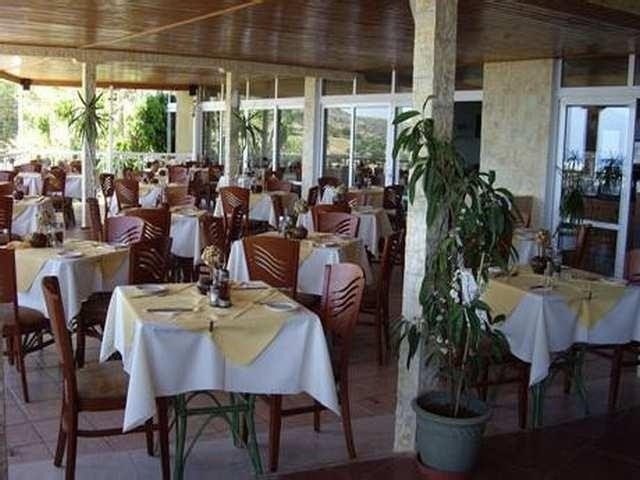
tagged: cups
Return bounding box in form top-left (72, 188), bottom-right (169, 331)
top-left (581, 281), bottom-right (592, 300)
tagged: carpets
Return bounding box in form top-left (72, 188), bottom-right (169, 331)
top-left (261, 405), bottom-right (640, 480)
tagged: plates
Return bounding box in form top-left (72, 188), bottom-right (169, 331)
top-left (103, 241), bottom-right (125, 249)
top-left (136, 284), bottom-right (165, 296)
top-left (55, 250), bottom-right (83, 260)
top-left (319, 240), bottom-right (335, 247)
top-left (265, 300), bottom-right (300, 312)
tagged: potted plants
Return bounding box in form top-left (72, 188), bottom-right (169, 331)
top-left (553, 150), bottom-right (592, 250)
top-left (391, 92), bottom-right (525, 473)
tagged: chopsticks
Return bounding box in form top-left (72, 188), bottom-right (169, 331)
top-left (231, 286), bottom-right (269, 291)
top-left (146, 307), bottom-right (192, 314)
